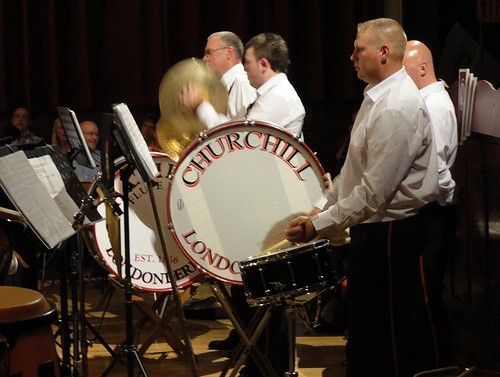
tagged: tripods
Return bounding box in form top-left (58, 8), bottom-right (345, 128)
top-left (16, 103), bottom-right (162, 377)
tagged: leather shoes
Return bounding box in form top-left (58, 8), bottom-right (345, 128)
top-left (208, 329), bottom-right (238, 349)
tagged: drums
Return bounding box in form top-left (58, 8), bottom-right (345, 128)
top-left (166, 119), bottom-right (333, 287)
top-left (85, 149), bottom-right (204, 292)
top-left (0, 286), bottom-right (61, 377)
top-left (238, 238), bottom-right (340, 309)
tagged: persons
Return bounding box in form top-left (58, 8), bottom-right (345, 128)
top-left (178, 31), bottom-right (259, 320)
top-left (208, 32), bottom-right (307, 376)
top-left (284, 18), bottom-right (432, 377)
top-left (405, 39), bottom-right (459, 377)
top-left (0, 98), bottom-right (176, 294)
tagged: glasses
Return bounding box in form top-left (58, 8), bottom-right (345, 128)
top-left (205, 46), bottom-right (230, 55)
top-left (82, 132), bottom-right (100, 137)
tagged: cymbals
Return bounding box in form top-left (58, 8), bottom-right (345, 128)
top-left (159, 57), bottom-right (228, 132)
top-left (156, 118), bottom-right (196, 162)
